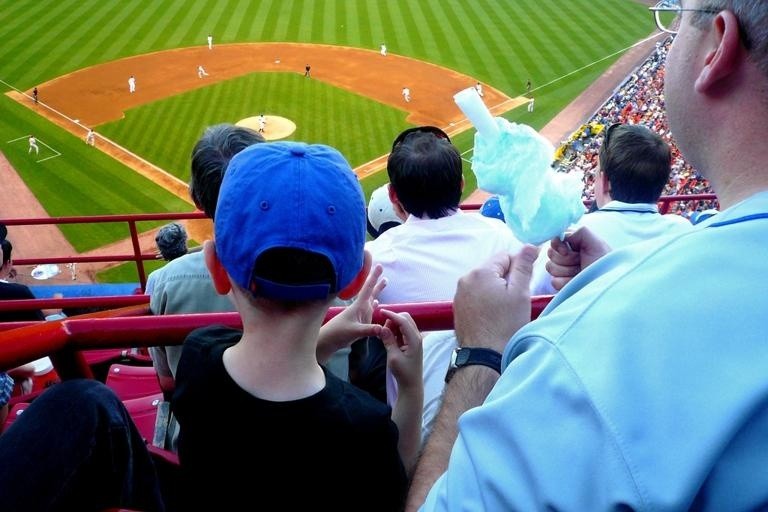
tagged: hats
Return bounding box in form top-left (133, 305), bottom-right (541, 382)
top-left (480, 196), bottom-right (506, 223)
top-left (154, 224), bottom-right (188, 258)
top-left (214, 142), bottom-right (366, 300)
top-left (367, 182), bottom-right (408, 238)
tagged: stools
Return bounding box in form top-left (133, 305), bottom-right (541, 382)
top-left (0, 349), bottom-right (182, 467)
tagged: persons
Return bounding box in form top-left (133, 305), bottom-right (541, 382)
top-left (528, 96), bottom-right (535, 112)
top-left (476, 82), bottom-right (483, 96)
top-left (379, 43), bottom-right (388, 57)
top-left (32, 87), bottom-right (40, 105)
top-left (196, 66), bottom-right (209, 81)
top-left (128, 75), bottom-right (136, 93)
top-left (406, 2), bottom-right (768, 508)
top-left (526, 78), bottom-right (533, 92)
top-left (304, 64), bottom-right (312, 79)
top-left (581, 37), bottom-right (675, 123)
top-left (2, 123), bottom-right (721, 510)
top-left (207, 34), bottom-right (213, 50)
top-left (257, 114), bottom-right (267, 134)
top-left (401, 87), bottom-right (411, 103)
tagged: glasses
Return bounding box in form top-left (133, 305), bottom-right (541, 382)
top-left (648, 1), bottom-right (720, 34)
top-left (393, 125), bottom-right (450, 155)
top-left (605, 123), bottom-right (622, 147)
